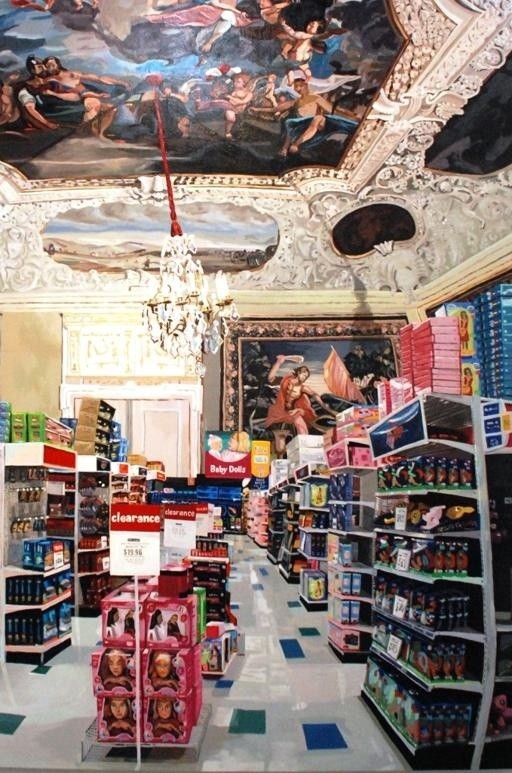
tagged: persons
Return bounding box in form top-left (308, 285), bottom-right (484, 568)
top-left (462, 367), bottom-right (474, 395)
top-left (124, 609), bottom-right (135, 638)
top-left (150, 697), bottom-right (183, 738)
top-left (147, 609), bottom-right (163, 641)
top-left (459, 309), bottom-right (470, 352)
top-left (166, 614), bottom-right (182, 642)
top-left (147, 648), bottom-right (181, 690)
top-left (106, 607), bottom-right (119, 638)
top-left (265, 353), bottom-right (339, 438)
top-left (104, 698), bottom-right (135, 736)
top-left (99, 649), bottom-right (136, 689)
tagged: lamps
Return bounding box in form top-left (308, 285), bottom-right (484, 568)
top-left (138, 96), bottom-right (239, 381)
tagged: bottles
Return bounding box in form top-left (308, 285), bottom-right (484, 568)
top-left (314, 581), bottom-right (321, 597)
top-left (316, 486), bottom-right (323, 503)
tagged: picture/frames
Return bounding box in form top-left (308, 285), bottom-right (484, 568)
top-left (220, 315), bottom-right (411, 454)
